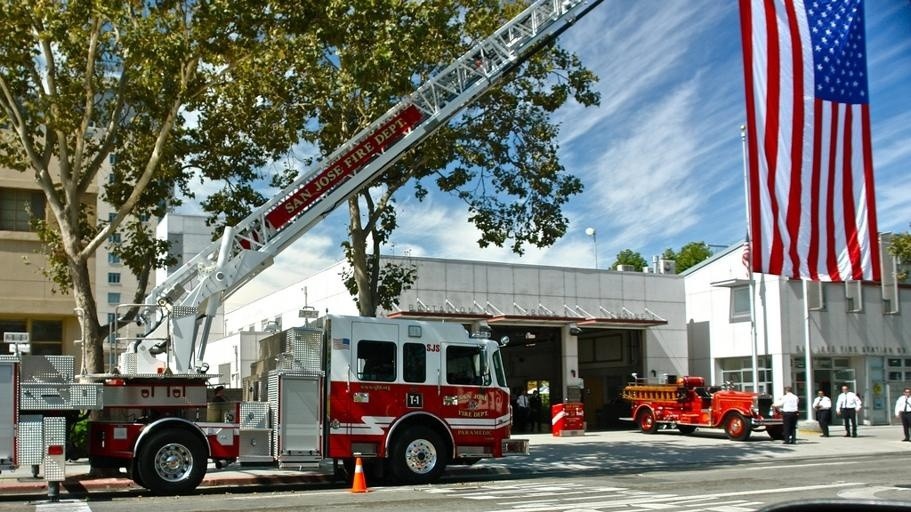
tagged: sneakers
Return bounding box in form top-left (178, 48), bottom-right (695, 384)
top-left (819, 434), bottom-right (829, 437)
top-left (901, 439), bottom-right (909, 442)
top-left (844, 434), bottom-right (857, 437)
top-left (783, 440), bottom-right (796, 444)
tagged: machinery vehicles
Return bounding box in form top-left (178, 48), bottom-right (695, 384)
top-left (614, 370), bottom-right (788, 444)
top-left (0, 0), bottom-right (603, 506)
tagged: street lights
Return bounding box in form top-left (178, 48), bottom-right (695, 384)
top-left (584, 226), bottom-right (599, 269)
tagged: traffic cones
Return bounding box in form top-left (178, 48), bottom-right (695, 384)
top-left (344, 457), bottom-right (369, 495)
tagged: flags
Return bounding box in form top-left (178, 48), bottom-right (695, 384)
top-left (738, 0), bottom-right (886, 287)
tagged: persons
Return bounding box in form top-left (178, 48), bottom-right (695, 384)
top-left (516, 389), bottom-right (532, 433)
top-left (836, 385), bottom-right (863, 437)
top-left (814, 389), bottom-right (833, 436)
top-left (772, 386), bottom-right (801, 444)
top-left (895, 387), bottom-right (911, 443)
top-left (210, 384), bottom-right (233, 422)
top-left (527, 389), bottom-right (544, 434)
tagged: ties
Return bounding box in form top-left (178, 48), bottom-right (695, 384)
top-left (817, 399), bottom-right (822, 407)
top-left (845, 394), bottom-right (848, 409)
top-left (523, 395), bottom-right (527, 407)
top-left (904, 398), bottom-right (911, 413)
